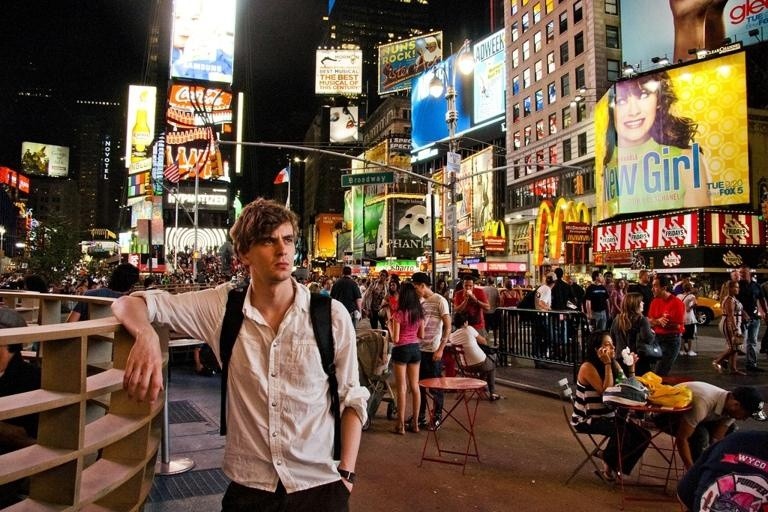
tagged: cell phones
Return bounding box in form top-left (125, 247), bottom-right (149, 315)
top-left (597, 346), bottom-right (606, 353)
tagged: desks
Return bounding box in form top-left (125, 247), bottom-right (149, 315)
top-left (418, 376), bottom-right (488, 475)
top-left (621, 401), bottom-right (696, 501)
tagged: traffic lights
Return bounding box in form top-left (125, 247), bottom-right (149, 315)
top-left (209, 149), bottom-right (224, 178)
top-left (144, 182), bottom-right (155, 202)
top-left (573, 174), bottom-right (583, 196)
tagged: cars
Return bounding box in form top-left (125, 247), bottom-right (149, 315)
top-left (497, 287), bottom-right (536, 303)
top-left (691, 292), bottom-right (723, 326)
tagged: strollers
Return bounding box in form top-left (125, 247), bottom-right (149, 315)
top-left (353, 327), bottom-right (402, 434)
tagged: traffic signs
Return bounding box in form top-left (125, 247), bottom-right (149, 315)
top-left (340, 171), bottom-right (393, 186)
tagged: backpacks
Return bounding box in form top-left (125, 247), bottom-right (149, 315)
top-left (519, 286), bottom-right (540, 322)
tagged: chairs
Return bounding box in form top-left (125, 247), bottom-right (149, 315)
top-left (556, 376), bottom-right (619, 489)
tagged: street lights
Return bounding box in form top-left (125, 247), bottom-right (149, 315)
top-left (429, 39), bottom-right (475, 288)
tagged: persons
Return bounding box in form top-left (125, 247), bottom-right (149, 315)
top-left (171, 0), bottom-right (233, 81)
top-left (415, 36), bottom-right (441, 65)
top-left (330, 107), bottom-right (357, 142)
top-left (112, 196), bottom-right (371, 512)
top-left (603, 71), bottom-right (711, 214)
top-left (0, 238), bottom-right (250, 376)
top-left (0, 306), bottom-right (41, 509)
top-left (291, 257), bottom-right (768, 512)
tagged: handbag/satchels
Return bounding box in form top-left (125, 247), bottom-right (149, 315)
top-left (732, 334), bottom-right (744, 344)
top-left (479, 344), bottom-right (497, 354)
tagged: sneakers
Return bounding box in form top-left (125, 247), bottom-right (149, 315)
top-left (390, 415), bottom-right (442, 434)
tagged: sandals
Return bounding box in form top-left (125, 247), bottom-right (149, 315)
top-left (491, 394), bottom-right (507, 400)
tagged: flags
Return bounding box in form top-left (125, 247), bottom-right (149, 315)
top-left (272, 165), bottom-right (289, 184)
top-left (162, 160), bottom-right (180, 184)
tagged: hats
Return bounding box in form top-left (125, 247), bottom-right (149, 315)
top-left (546, 272), bottom-right (557, 281)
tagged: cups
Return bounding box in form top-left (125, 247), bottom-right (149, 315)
top-left (664, 314), bottom-right (672, 327)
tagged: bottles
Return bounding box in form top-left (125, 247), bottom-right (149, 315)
top-left (615, 369), bottom-right (625, 385)
